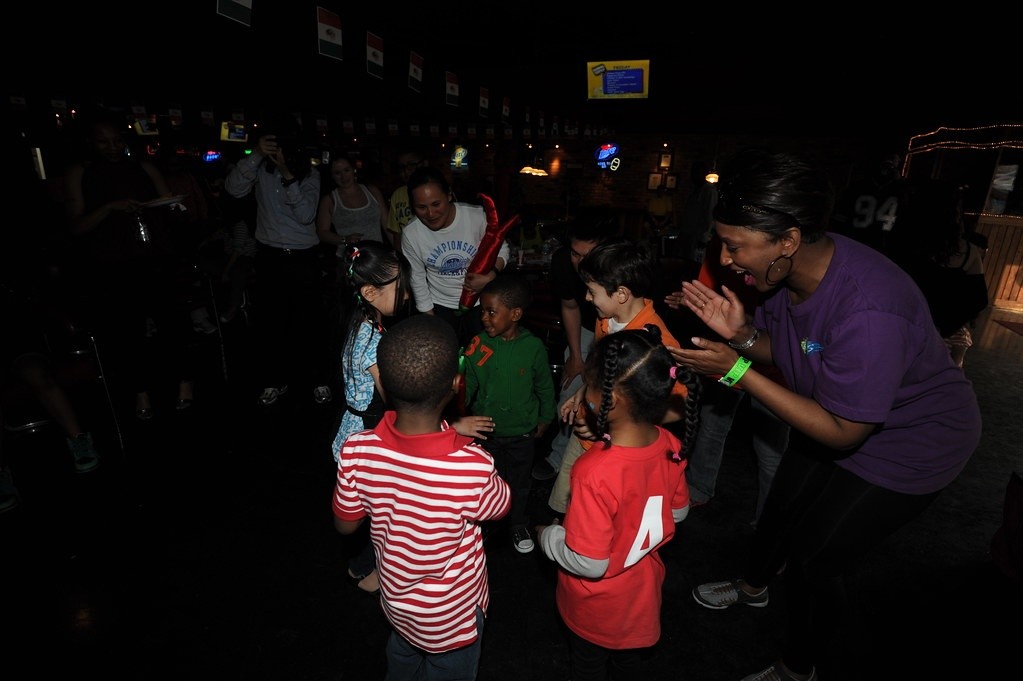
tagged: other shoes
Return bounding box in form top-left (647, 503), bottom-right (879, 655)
top-left (223, 291), bottom-right (238, 312)
top-left (193, 319), bottom-right (216, 335)
top-left (219, 309), bottom-right (237, 323)
top-left (689, 485), bottom-right (710, 510)
top-left (358, 569), bottom-right (381, 596)
top-left (143, 318), bottom-right (157, 338)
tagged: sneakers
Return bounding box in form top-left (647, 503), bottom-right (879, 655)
top-left (66, 431), bottom-right (100, 473)
top-left (312, 384), bottom-right (332, 404)
top-left (257, 384), bottom-right (288, 408)
top-left (510, 526), bottom-right (535, 554)
top-left (741, 659), bottom-right (818, 681)
top-left (0, 465), bottom-right (19, 514)
top-left (692, 578), bottom-right (769, 610)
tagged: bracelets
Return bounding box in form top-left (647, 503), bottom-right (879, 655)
top-left (718, 356), bottom-right (753, 388)
top-left (729, 325), bottom-right (759, 350)
top-left (340, 236), bottom-right (346, 244)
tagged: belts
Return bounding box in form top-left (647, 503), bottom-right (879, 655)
top-left (256, 243), bottom-right (319, 256)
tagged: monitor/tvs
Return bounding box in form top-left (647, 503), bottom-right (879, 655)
top-left (586, 58), bottom-right (650, 100)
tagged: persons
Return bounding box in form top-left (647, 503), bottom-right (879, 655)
top-left (669, 149), bottom-right (982, 681)
top-left (0, 107), bottom-right (989, 681)
top-left (332, 315), bottom-right (512, 681)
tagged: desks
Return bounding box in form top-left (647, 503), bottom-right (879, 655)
top-left (506, 254), bottom-right (596, 352)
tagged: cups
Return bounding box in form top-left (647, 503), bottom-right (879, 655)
top-left (516, 250), bottom-right (527, 267)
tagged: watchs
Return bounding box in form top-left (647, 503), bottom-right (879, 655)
top-left (280, 176), bottom-right (297, 187)
